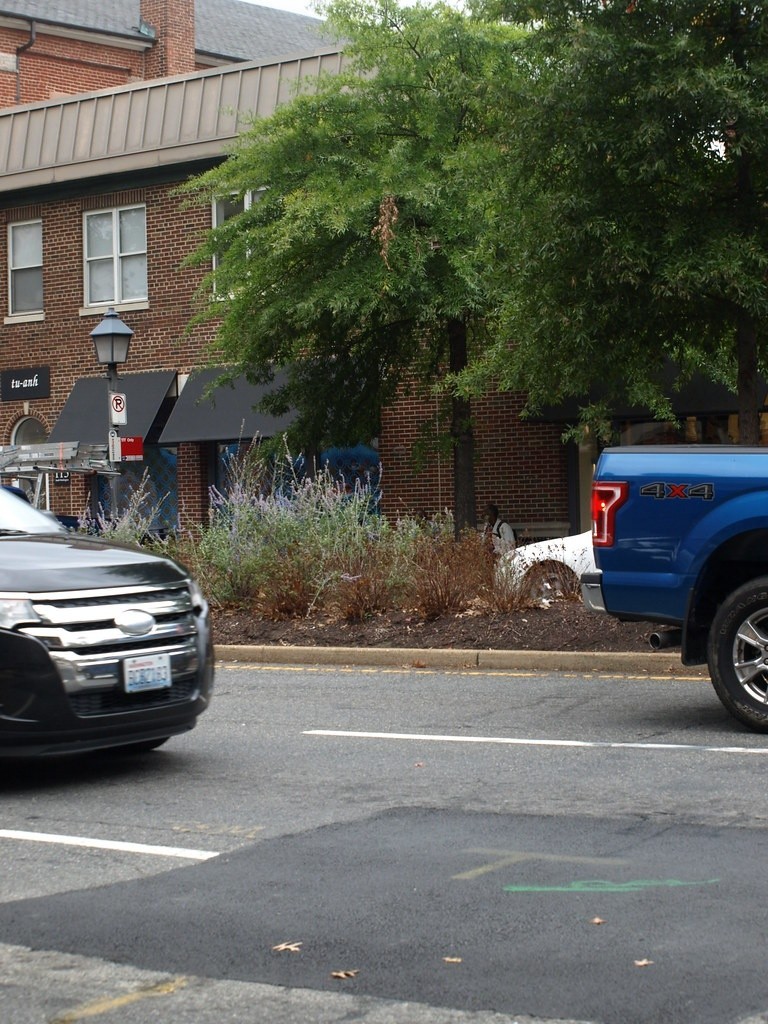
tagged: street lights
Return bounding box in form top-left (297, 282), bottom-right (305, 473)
top-left (90, 308), bottom-right (134, 528)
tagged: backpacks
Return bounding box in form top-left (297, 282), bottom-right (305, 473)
top-left (494, 520), bottom-right (518, 548)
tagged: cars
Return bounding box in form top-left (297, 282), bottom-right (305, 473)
top-left (0, 487), bottom-right (214, 756)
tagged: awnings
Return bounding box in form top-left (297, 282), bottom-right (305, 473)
top-left (518, 309), bottom-right (768, 423)
top-left (45, 370), bottom-right (177, 443)
top-left (158, 355), bottom-right (376, 449)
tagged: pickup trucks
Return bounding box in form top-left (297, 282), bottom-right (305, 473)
top-left (590, 445), bottom-right (768, 731)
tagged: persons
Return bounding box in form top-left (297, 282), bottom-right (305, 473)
top-left (404, 509), bottom-right (445, 540)
top-left (480, 505), bottom-right (517, 556)
